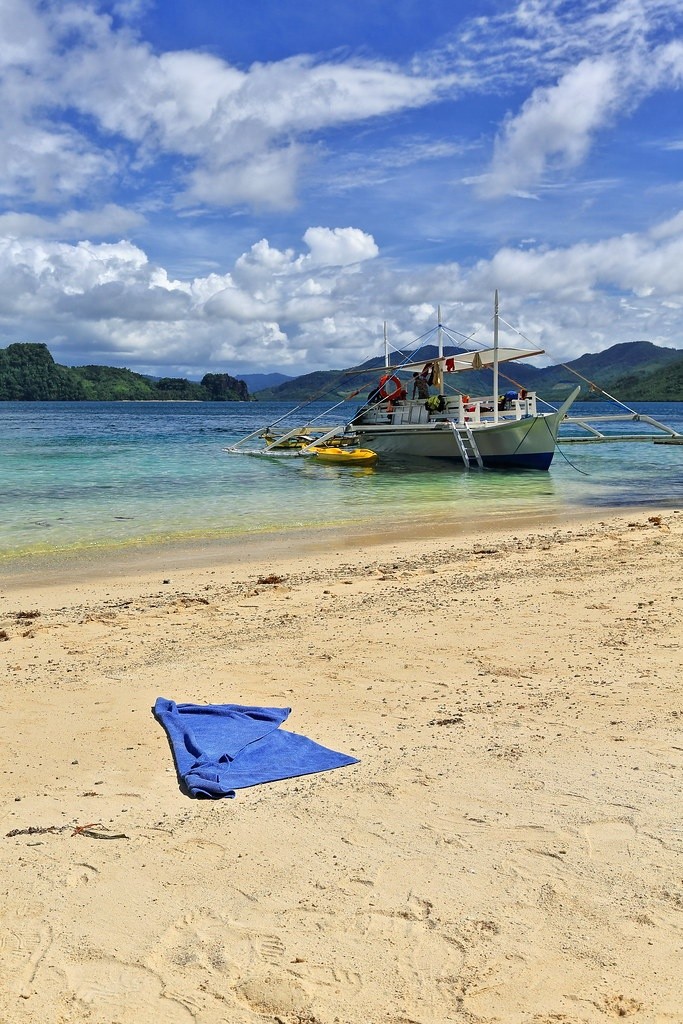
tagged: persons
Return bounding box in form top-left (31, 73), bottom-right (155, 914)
top-left (412, 372), bottom-right (430, 399)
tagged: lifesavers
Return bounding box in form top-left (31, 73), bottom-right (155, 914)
top-left (378, 375), bottom-right (402, 400)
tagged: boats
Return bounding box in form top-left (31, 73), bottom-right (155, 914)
top-left (223, 288), bottom-right (683, 471)
top-left (301, 442), bottom-right (378, 462)
top-left (259, 429), bottom-right (360, 447)
